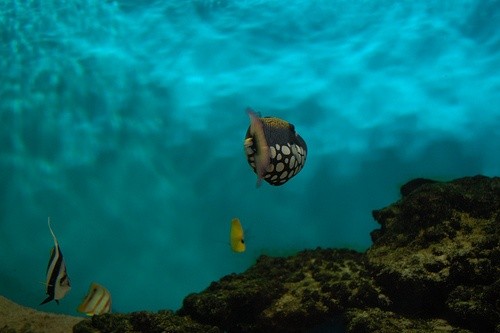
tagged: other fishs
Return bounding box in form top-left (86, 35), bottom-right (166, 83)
top-left (230, 217), bottom-right (246, 253)
top-left (38, 217), bottom-right (70, 306)
top-left (244, 108), bottom-right (307, 189)
top-left (78, 283), bottom-right (112, 317)
